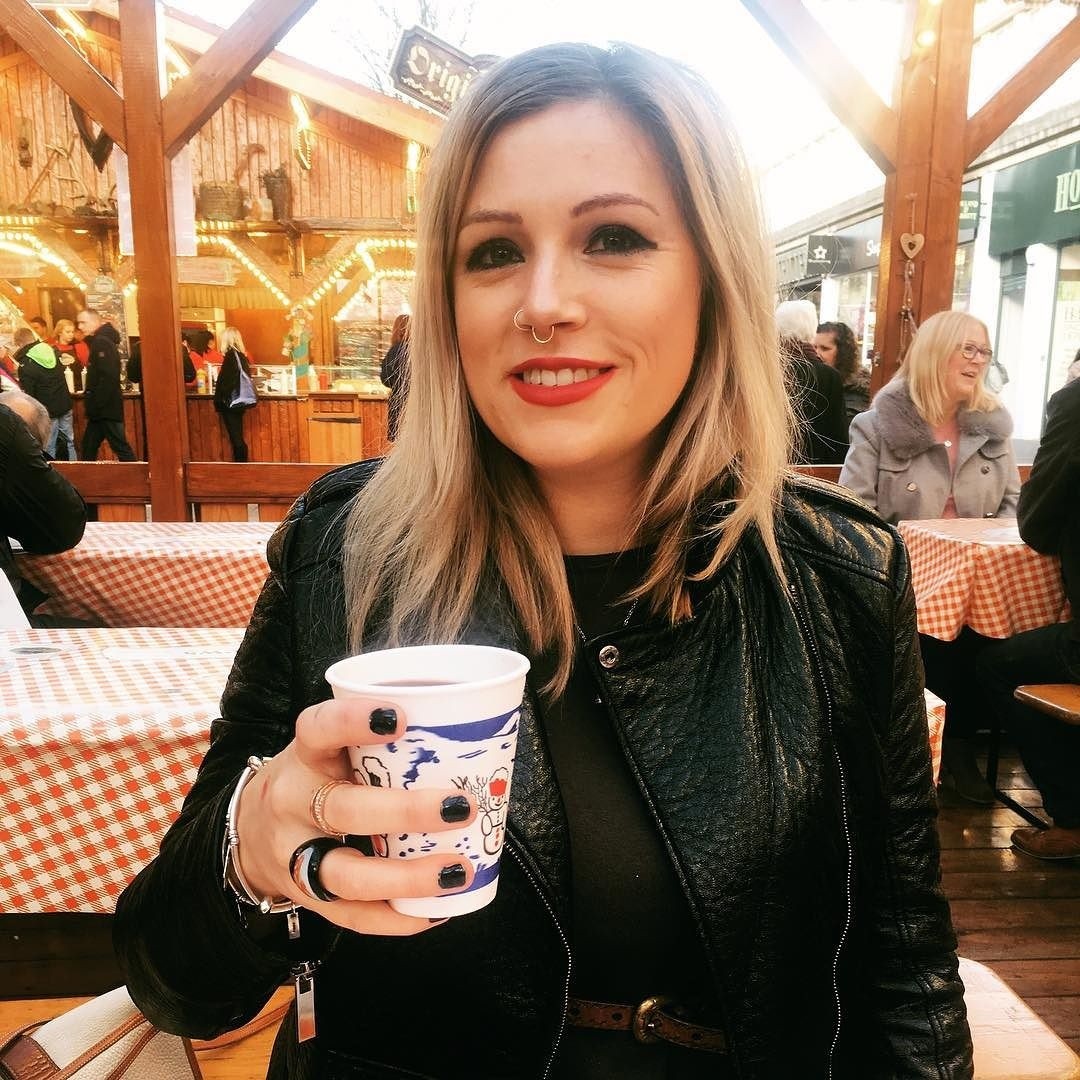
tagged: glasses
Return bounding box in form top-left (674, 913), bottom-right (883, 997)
top-left (954, 343), bottom-right (992, 363)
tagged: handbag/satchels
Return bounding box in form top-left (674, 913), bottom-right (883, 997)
top-left (0, 985), bottom-right (204, 1080)
top-left (222, 350), bottom-right (258, 410)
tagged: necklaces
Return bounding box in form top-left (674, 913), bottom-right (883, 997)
top-left (944, 439), bottom-right (953, 448)
top-left (575, 596), bottom-right (638, 703)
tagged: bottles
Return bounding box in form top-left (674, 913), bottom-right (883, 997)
top-left (64, 366), bottom-right (74, 393)
top-left (280, 369), bottom-right (288, 394)
top-left (320, 369), bottom-right (327, 390)
top-left (81, 366), bottom-right (87, 391)
top-left (197, 366), bottom-right (206, 394)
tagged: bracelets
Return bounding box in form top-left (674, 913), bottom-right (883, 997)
top-left (222, 755), bottom-right (304, 942)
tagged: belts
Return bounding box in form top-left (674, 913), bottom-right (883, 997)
top-left (492, 988), bottom-right (727, 1053)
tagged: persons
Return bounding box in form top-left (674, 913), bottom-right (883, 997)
top-left (775, 299), bottom-right (872, 464)
top-left (380, 314), bottom-right (410, 442)
top-left (78, 308), bottom-right (137, 463)
top-left (0, 390), bottom-right (111, 629)
top-left (111, 38), bottom-right (978, 1080)
top-left (213, 326), bottom-right (251, 463)
top-left (0, 317), bottom-right (90, 461)
top-left (125, 331), bottom-right (225, 463)
top-left (975, 361), bottom-right (1080, 859)
top-left (838, 310), bottom-right (1023, 804)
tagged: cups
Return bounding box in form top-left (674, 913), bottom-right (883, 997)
top-left (325, 645), bottom-right (531, 920)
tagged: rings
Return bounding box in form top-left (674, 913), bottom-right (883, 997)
top-left (308, 777), bottom-right (353, 837)
top-left (288, 837), bottom-right (348, 903)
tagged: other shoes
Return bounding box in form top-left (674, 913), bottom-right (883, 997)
top-left (939, 765), bottom-right (995, 803)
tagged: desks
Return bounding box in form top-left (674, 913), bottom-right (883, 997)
top-left (0, 521), bottom-right (1080, 1080)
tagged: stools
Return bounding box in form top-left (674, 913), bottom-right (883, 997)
top-left (1014, 683), bottom-right (1080, 726)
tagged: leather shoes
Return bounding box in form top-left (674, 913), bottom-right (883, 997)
top-left (1011, 823), bottom-right (1080, 859)
